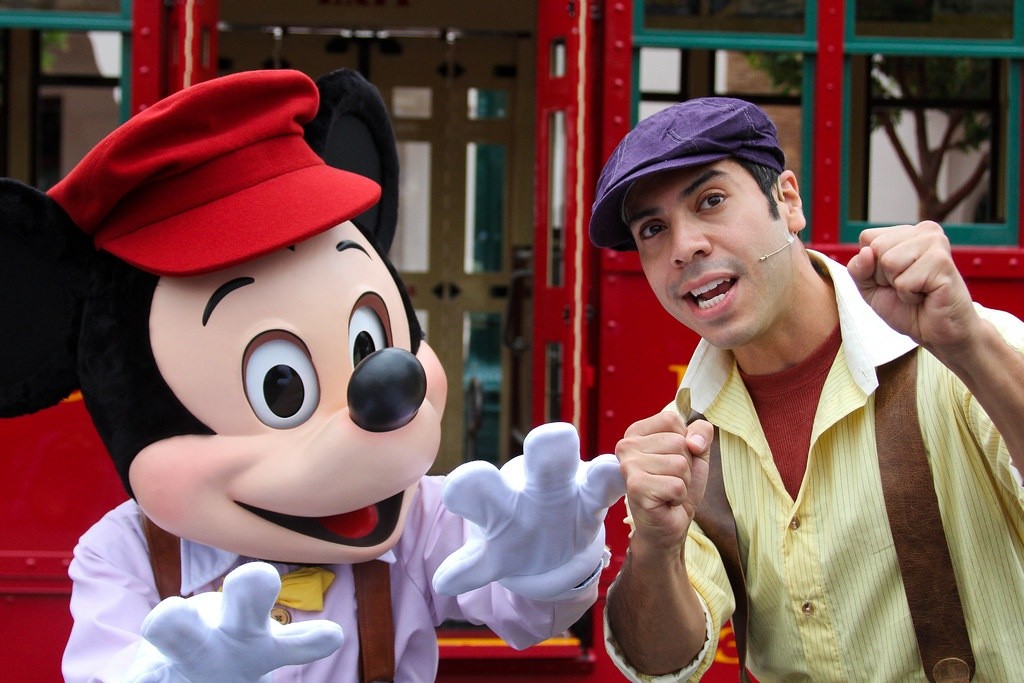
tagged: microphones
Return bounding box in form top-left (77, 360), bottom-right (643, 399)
top-left (760, 243), bottom-right (790, 261)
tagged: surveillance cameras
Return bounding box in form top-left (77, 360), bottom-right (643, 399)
top-left (429, 50), bottom-right (471, 82)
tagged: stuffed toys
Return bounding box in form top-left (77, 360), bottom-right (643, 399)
top-left (0, 68), bottom-right (627, 683)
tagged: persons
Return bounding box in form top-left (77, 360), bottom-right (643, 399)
top-left (590, 98), bottom-right (1024, 683)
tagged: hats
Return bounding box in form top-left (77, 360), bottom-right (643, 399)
top-left (589, 97), bottom-right (785, 252)
